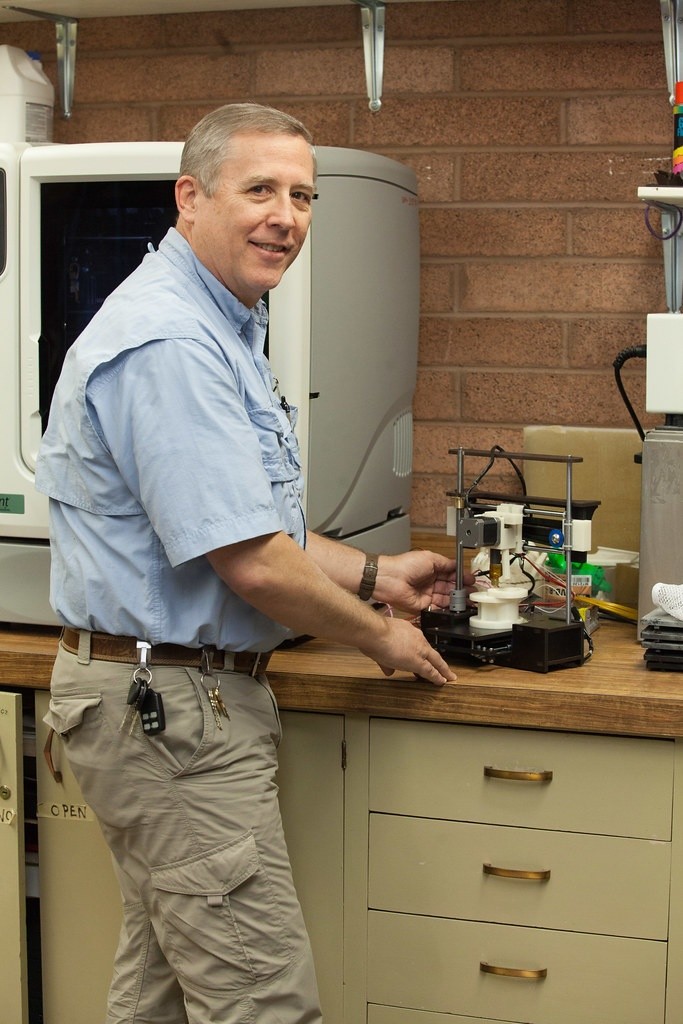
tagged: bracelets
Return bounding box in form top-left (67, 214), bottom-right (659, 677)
top-left (356, 551), bottom-right (379, 601)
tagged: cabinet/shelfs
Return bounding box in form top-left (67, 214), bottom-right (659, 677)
top-left (0, 532), bottom-right (683, 1024)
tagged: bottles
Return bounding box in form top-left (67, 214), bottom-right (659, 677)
top-left (672, 81), bottom-right (683, 180)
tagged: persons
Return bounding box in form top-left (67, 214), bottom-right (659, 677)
top-left (35, 99), bottom-right (481, 1024)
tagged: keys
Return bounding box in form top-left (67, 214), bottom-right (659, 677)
top-left (207, 687), bottom-right (231, 731)
top-left (117, 677), bottom-right (166, 738)
